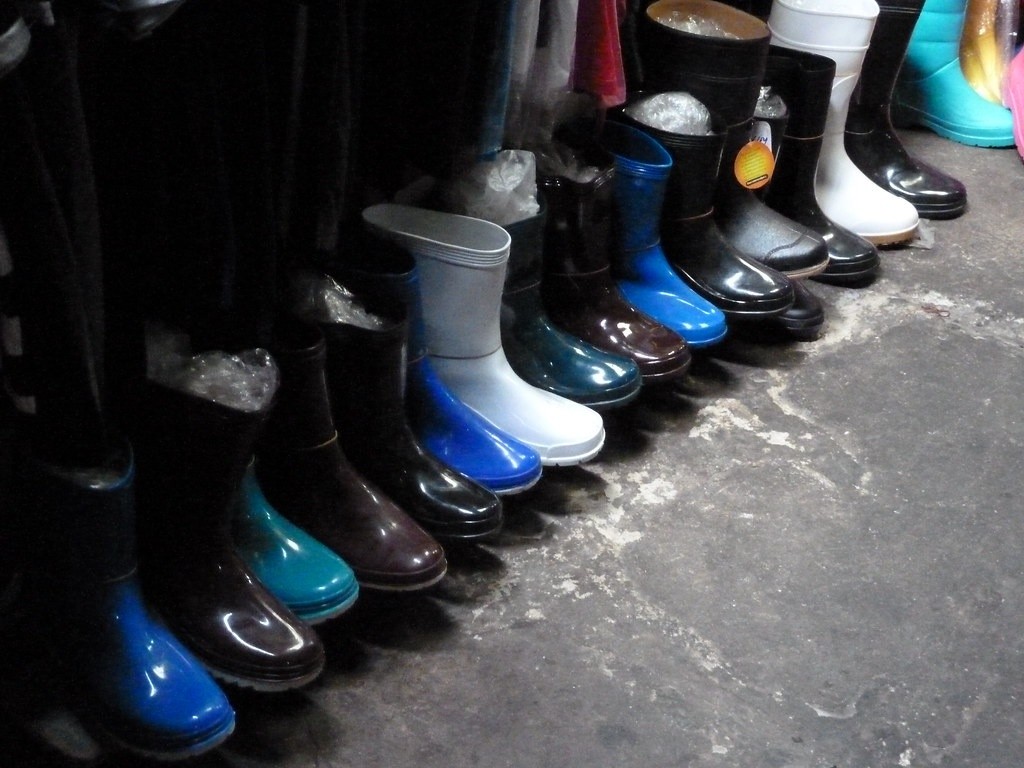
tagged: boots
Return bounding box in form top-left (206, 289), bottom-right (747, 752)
top-left (53, 430), bottom-right (236, 763)
top-left (111, 0), bottom-right (1023, 693)
